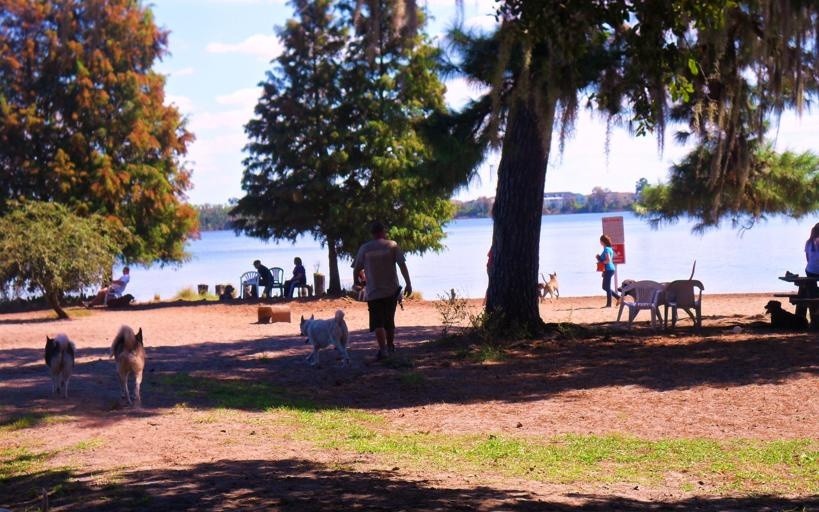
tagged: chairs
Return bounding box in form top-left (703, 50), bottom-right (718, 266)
top-left (104, 284), bottom-right (126, 304)
top-left (616, 279), bottom-right (705, 331)
top-left (239, 267), bottom-right (284, 298)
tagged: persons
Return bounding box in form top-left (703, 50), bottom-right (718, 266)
top-left (278, 257), bottom-right (307, 301)
top-left (253, 259), bottom-right (276, 300)
top-left (81, 266), bottom-right (130, 309)
top-left (595, 234), bottom-right (623, 308)
top-left (352, 269), bottom-right (367, 302)
top-left (803, 222), bottom-right (819, 328)
top-left (353, 220), bottom-right (413, 361)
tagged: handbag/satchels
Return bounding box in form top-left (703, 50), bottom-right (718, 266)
top-left (597, 261), bottom-right (606, 272)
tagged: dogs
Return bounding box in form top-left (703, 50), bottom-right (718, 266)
top-left (108, 325), bottom-right (145, 406)
top-left (43, 332), bottom-right (75, 399)
top-left (219, 284), bottom-right (235, 301)
top-left (299, 309), bottom-right (351, 369)
top-left (105, 293), bottom-right (135, 307)
top-left (616, 259), bottom-right (698, 328)
top-left (537, 272), bottom-right (560, 303)
top-left (764, 300), bottom-right (808, 332)
